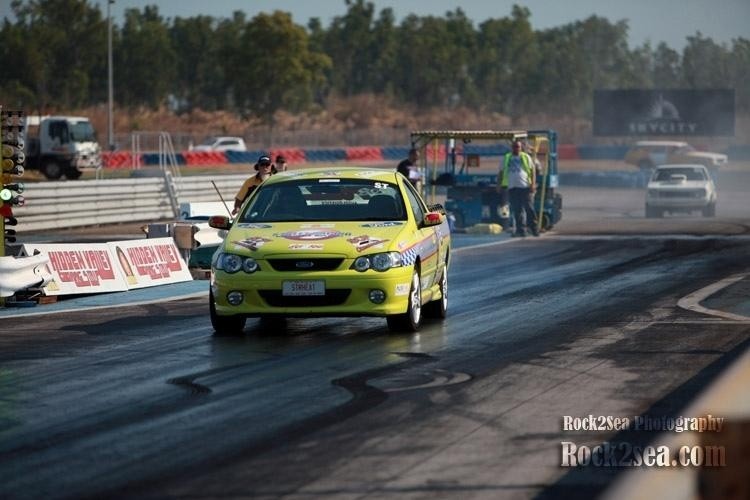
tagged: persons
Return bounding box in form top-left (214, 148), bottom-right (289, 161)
top-left (499, 142), bottom-right (543, 239)
top-left (396, 149), bottom-right (421, 192)
top-left (230, 153), bottom-right (288, 213)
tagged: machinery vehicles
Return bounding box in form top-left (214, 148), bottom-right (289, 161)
top-left (410, 128), bottom-right (560, 231)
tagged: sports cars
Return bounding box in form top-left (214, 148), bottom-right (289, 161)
top-left (206, 166), bottom-right (452, 333)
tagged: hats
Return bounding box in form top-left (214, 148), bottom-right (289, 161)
top-left (258, 155), bottom-right (271, 164)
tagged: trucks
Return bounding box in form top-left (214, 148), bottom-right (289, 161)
top-left (21, 112), bottom-right (102, 181)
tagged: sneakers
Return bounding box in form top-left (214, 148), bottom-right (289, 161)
top-left (511, 230), bottom-right (540, 238)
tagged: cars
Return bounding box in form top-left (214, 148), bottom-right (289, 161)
top-left (626, 139), bottom-right (728, 169)
top-left (193, 136), bottom-right (249, 155)
top-left (644, 163), bottom-right (719, 219)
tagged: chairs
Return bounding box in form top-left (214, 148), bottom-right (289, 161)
top-left (262, 185), bottom-right (400, 219)
